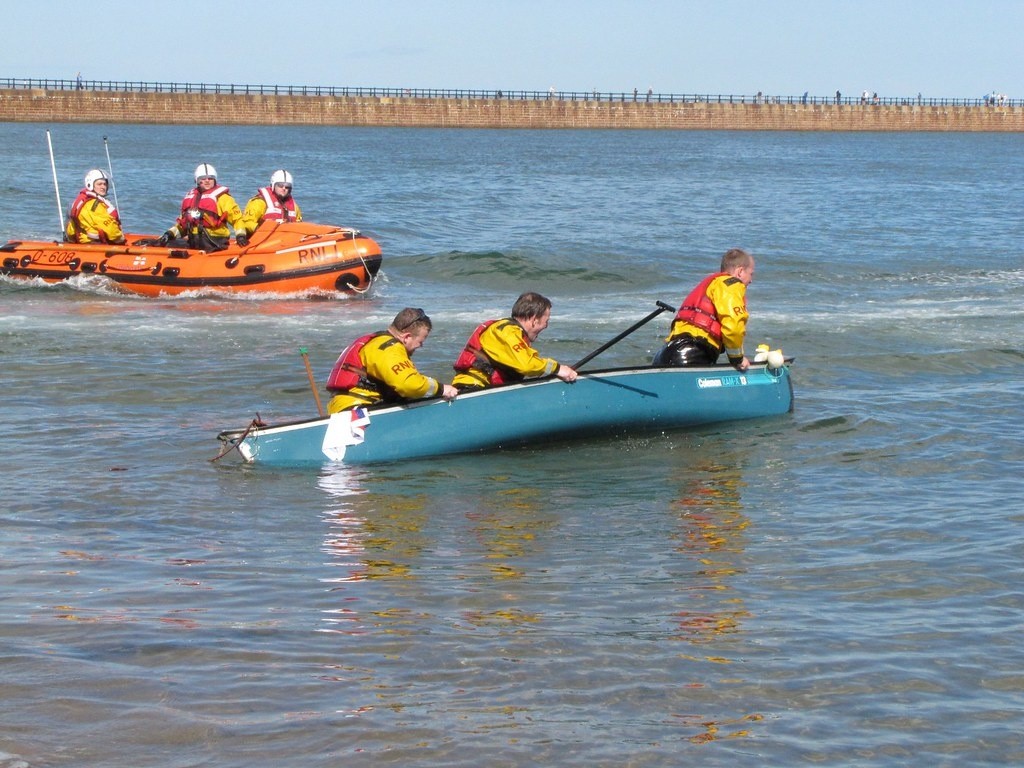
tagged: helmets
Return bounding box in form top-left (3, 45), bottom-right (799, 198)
top-left (269, 169), bottom-right (294, 193)
top-left (85, 169), bottom-right (111, 191)
top-left (194, 163), bottom-right (217, 185)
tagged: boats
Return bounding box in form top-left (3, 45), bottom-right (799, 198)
top-left (1, 207), bottom-right (383, 312)
top-left (216, 352), bottom-right (800, 475)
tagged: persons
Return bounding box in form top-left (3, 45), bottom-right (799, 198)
top-left (63, 169), bottom-right (128, 247)
top-left (452, 290), bottom-right (583, 393)
top-left (242, 170), bottom-right (302, 238)
top-left (324, 305), bottom-right (463, 418)
top-left (156, 161), bottom-right (250, 253)
top-left (651, 247), bottom-right (753, 373)
top-left (746, 89), bottom-right (1017, 108)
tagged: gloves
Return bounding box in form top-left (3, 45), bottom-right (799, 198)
top-left (235, 235), bottom-right (250, 246)
top-left (155, 233), bottom-right (168, 244)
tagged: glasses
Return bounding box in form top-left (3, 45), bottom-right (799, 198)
top-left (402, 308), bottom-right (424, 330)
top-left (276, 182), bottom-right (292, 190)
top-left (199, 177), bottom-right (215, 180)
